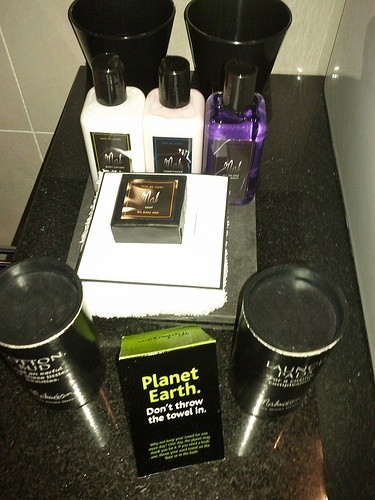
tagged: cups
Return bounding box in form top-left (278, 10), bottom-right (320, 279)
top-left (68, 0), bottom-right (176, 98)
top-left (183, 0), bottom-right (294, 100)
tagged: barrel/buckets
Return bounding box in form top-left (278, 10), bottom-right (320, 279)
top-left (0, 255), bottom-right (106, 412)
top-left (227, 263), bottom-right (349, 418)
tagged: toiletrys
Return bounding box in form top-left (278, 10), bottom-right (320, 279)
top-left (203, 60), bottom-right (268, 206)
top-left (145, 54), bottom-right (206, 174)
top-left (78, 51), bottom-right (146, 193)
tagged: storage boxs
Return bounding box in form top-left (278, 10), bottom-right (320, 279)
top-left (116, 325), bottom-right (226, 479)
top-left (111, 174), bottom-right (186, 244)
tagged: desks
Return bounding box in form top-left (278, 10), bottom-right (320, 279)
top-left (0, 65), bottom-right (375, 500)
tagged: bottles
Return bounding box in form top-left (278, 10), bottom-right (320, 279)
top-left (204, 58), bottom-right (268, 208)
top-left (79, 52), bottom-right (146, 193)
top-left (146, 55), bottom-right (206, 174)
